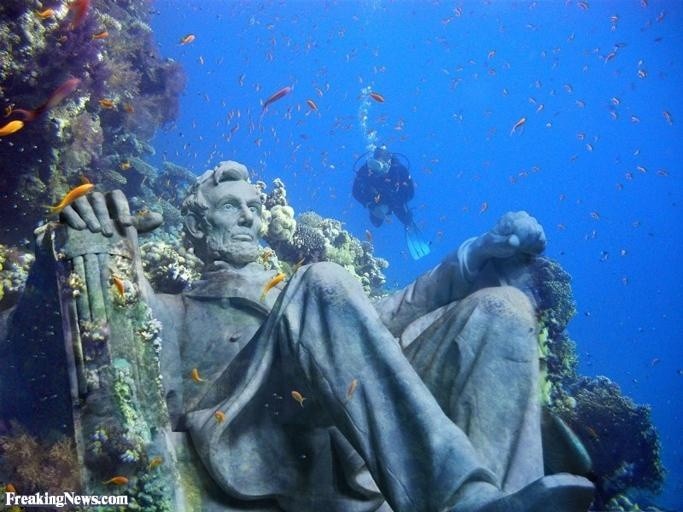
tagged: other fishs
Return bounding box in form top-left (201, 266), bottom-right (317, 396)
top-left (0, 0), bottom-right (682, 512)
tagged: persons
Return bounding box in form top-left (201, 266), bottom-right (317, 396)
top-left (352, 142), bottom-right (415, 228)
top-left (1, 158), bottom-right (598, 512)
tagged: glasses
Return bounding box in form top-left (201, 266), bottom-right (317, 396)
top-left (365, 159), bottom-right (384, 172)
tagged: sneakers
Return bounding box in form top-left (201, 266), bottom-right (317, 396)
top-left (405, 212), bottom-right (414, 228)
